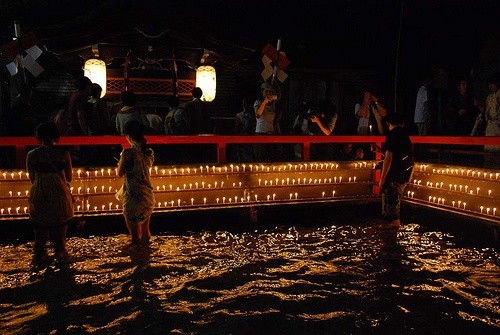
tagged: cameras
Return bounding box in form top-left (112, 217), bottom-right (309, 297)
top-left (270, 95), bottom-right (277, 100)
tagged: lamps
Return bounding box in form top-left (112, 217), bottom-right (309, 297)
top-left (83, 52), bottom-right (107, 98)
top-left (195, 57), bottom-right (217, 102)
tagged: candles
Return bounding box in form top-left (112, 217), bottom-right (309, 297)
top-left (0, 161), bottom-right (500, 216)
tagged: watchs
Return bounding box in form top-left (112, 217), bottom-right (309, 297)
top-left (375, 101), bottom-right (380, 104)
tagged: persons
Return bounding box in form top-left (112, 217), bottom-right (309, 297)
top-left (117, 119), bottom-right (157, 244)
top-left (0, 75), bottom-right (500, 171)
top-left (377, 111), bottom-right (413, 224)
top-left (25, 121), bottom-right (76, 265)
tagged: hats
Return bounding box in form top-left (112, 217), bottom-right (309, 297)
top-left (262, 83), bottom-right (272, 90)
